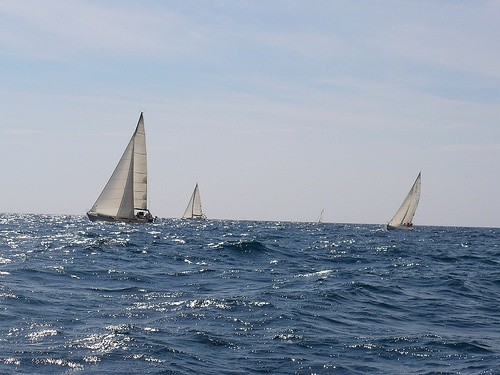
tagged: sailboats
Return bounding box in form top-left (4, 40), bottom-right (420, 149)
top-left (386, 171), bottom-right (421, 228)
top-left (88, 112), bottom-right (157, 222)
top-left (182, 184), bottom-right (203, 219)
